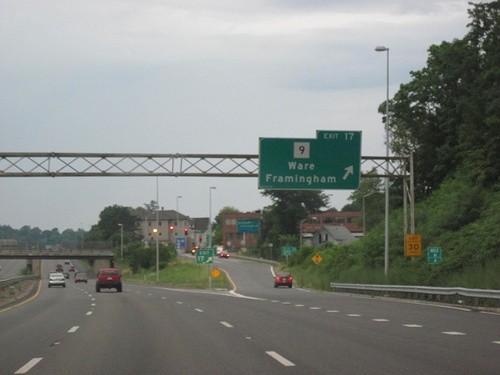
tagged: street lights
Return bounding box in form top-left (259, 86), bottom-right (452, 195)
top-left (118, 224), bottom-right (124, 261)
top-left (176, 195), bottom-right (183, 234)
top-left (209, 186), bottom-right (216, 248)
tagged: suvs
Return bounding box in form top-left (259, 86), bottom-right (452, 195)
top-left (47, 260), bottom-right (123, 293)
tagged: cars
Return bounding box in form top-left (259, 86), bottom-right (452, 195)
top-left (274, 273), bottom-right (293, 288)
top-left (192, 247), bottom-right (231, 260)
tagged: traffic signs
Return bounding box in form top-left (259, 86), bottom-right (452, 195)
top-left (258, 129), bottom-right (361, 190)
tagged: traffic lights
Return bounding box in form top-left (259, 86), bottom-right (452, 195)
top-left (169, 225), bottom-right (203, 242)
